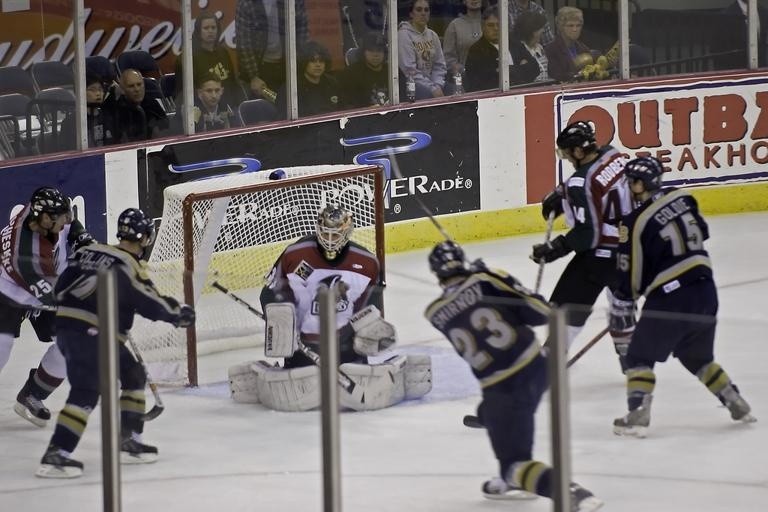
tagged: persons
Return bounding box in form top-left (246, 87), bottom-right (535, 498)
top-left (0, 184), bottom-right (100, 370)
top-left (260, 205), bottom-right (383, 369)
top-left (33, 208), bottom-right (196, 478)
top-left (59, 69), bottom-right (170, 154)
top-left (172, 1), bottom-right (656, 135)
top-left (423, 240), bottom-right (603, 512)
top-left (531, 120), bottom-right (643, 374)
top-left (608, 155), bottom-right (757, 438)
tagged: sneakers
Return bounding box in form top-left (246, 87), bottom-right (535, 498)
top-left (120, 430), bottom-right (157, 452)
top-left (41, 447), bottom-right (83, 469)
top-left (570, 483), bottom-right (592, 511)
top-left (483, 479), bottom-right (525, 494)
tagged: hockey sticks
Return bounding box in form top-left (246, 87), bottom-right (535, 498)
top-left (386, 146), bottom-right (474, 266)
top-left (213, 281), bottom-right (395, 404)
top-left (463, 325), bottom-right (609, 429)
top-left (125, 329), bottom-right (165, 422)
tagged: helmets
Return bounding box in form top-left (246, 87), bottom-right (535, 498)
top-left (554, 120), bottom-right (597, 160)
top-left (429, 241), bottom-right (466, 279)
top-left (30, 186), bottom-right (73, 217)
top-left (117, 208), bottom-right (156, 247)
top-left (315, 205), bottom-right (353, 259)
top-left (625, 157), bottom-right (663, 191)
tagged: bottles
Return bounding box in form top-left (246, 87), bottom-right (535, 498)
top-left (452, 67), bottom-right (463, 96)
top-left (405, 72), bottom-right (415, 103)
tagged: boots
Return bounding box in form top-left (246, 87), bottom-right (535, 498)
top-left (17, 367), bottom-right (50, 419)
top-left (722, 385), bottom-right (750, 420)
top-left (614, 392), bottom-right (654, 426)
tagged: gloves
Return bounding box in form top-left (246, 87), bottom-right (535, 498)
top-left (533, 235), bottom-right (573, 263)
top-left (542, 191), bottom-right (564, 220)
top-left (180, 304), bottom-right (195, 328)
